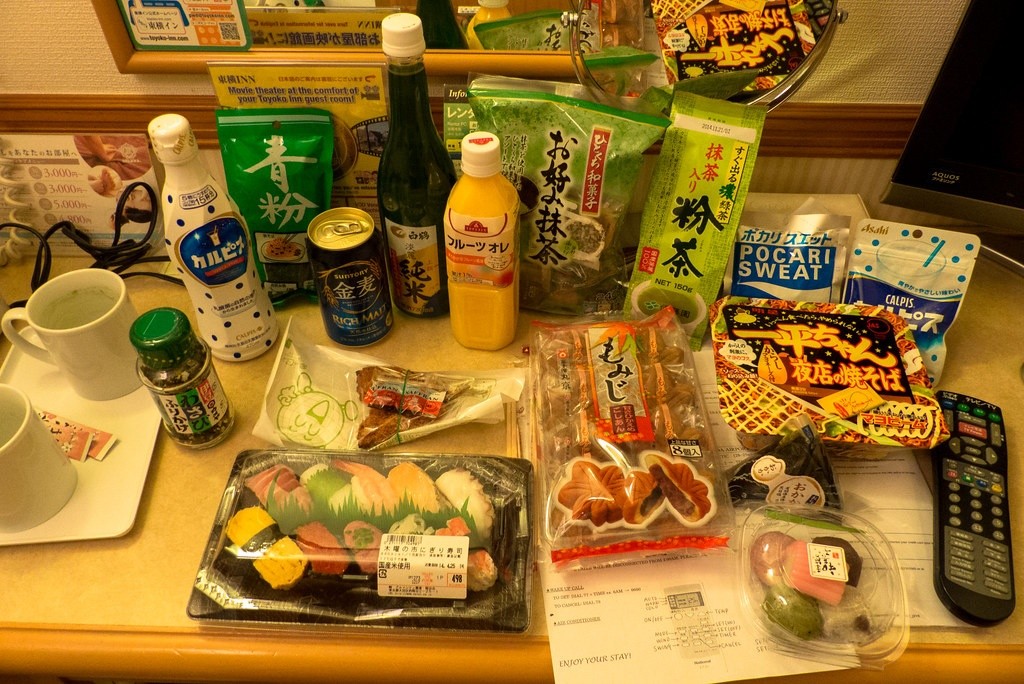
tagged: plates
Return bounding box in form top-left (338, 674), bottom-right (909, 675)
top-left (187, 450), bottom-right (533, 634)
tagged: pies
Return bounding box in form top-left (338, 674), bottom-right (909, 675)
top-left (556, 454), bottom-right (712, 526)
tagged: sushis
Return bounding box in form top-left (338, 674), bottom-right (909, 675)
top-left (222, 464), bottom-right (500, 593)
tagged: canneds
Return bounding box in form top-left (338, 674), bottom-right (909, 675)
top-left (305, 207), bottom-right (393, 348)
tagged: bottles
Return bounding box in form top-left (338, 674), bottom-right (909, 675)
top-left (442, 130), bottom-right (521, 352)
top-left (466, 0), bottom-right (512, 50)
top-left (147, 113), bottom-right (279, 363)
top-left (376, 12), bottom-right (450, 320)
top-left (129, 307), bottom-right (235, 450)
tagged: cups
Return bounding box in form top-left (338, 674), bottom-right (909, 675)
top-left (0, 383), bottom-right (79, 534)
top-left (0, 268), bottom-right (144, 401)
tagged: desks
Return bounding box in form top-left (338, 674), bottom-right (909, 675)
top-left (0, 252), bottom-right (1024, 684)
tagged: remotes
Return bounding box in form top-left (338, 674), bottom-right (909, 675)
top-left (932, 390), bottom-right (1017, 628)
